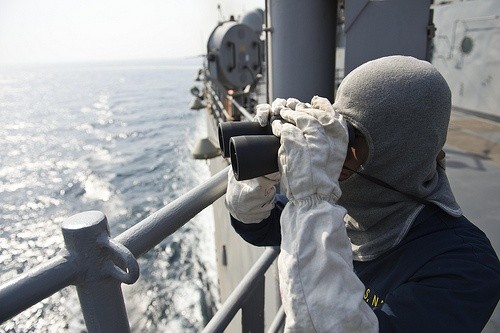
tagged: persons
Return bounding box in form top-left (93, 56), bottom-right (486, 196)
top-left (224, 56), bottom-right (500, 333)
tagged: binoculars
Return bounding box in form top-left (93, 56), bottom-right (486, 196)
top-left (217, 121), bottom-right (282, 182)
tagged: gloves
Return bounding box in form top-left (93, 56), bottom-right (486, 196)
top-left (271, 95), bottom-right (380, 333)
top-left (225, 98), bottom-right (313, 224)
